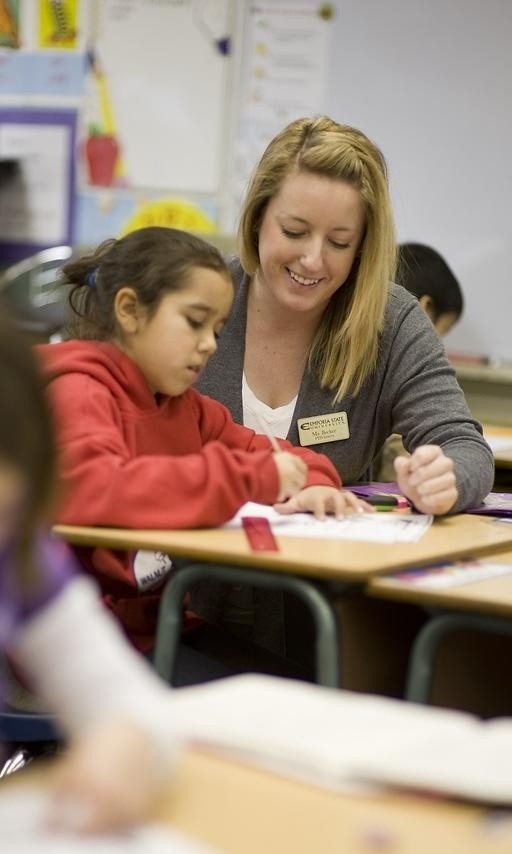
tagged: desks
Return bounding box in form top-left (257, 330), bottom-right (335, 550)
top-left (51, 491), bottom-right (512, 689)
top-left (474, 418), bottom-right (512, 471)
top-left (1, 670), bottom-right (512, 853)
top-left (367, 551), bottom-right (511, 711)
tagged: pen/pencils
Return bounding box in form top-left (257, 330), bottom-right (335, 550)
top-left (249, 406), bottom-right (282, 454)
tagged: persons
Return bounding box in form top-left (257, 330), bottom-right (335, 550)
top-left (389, 241), bottom-right (466, 340)
top-left (0, 293), bottom-right (184, 837)
top-left (191, 116), bottom-right (497, 517)
top-left (38, 228), bottom-right (375, 690)
